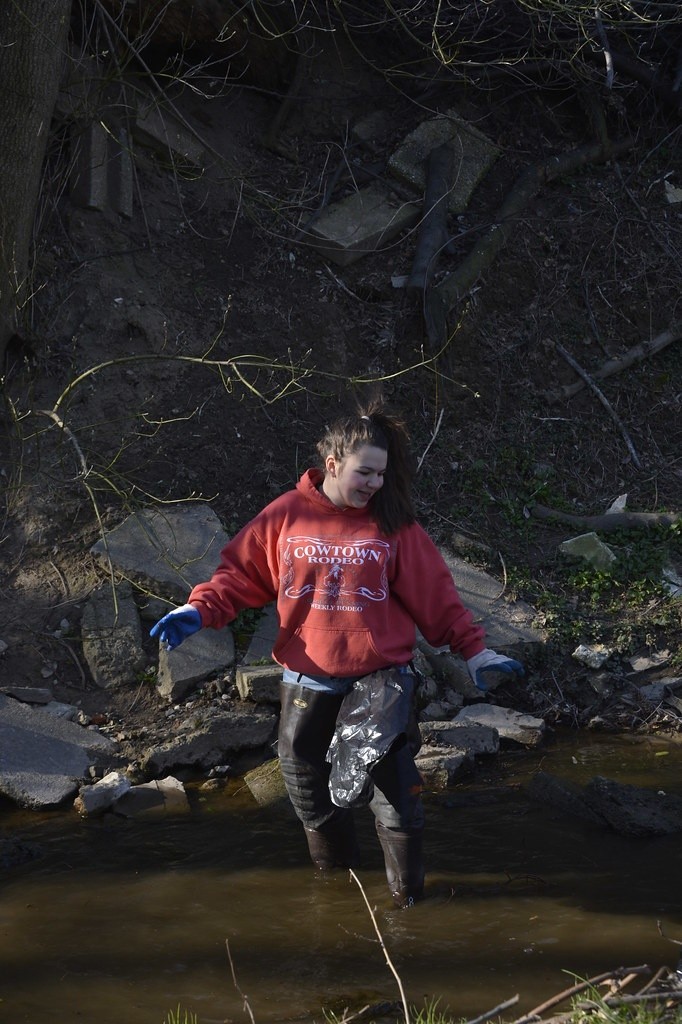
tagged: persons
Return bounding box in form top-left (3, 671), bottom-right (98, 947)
top-left (149, 392), bottom-right (525, 914)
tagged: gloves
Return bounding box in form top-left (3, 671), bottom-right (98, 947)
top-left (467, 648), bottom-right (526, 692)
top-left (150, 603), bottom-right (202, 653)
